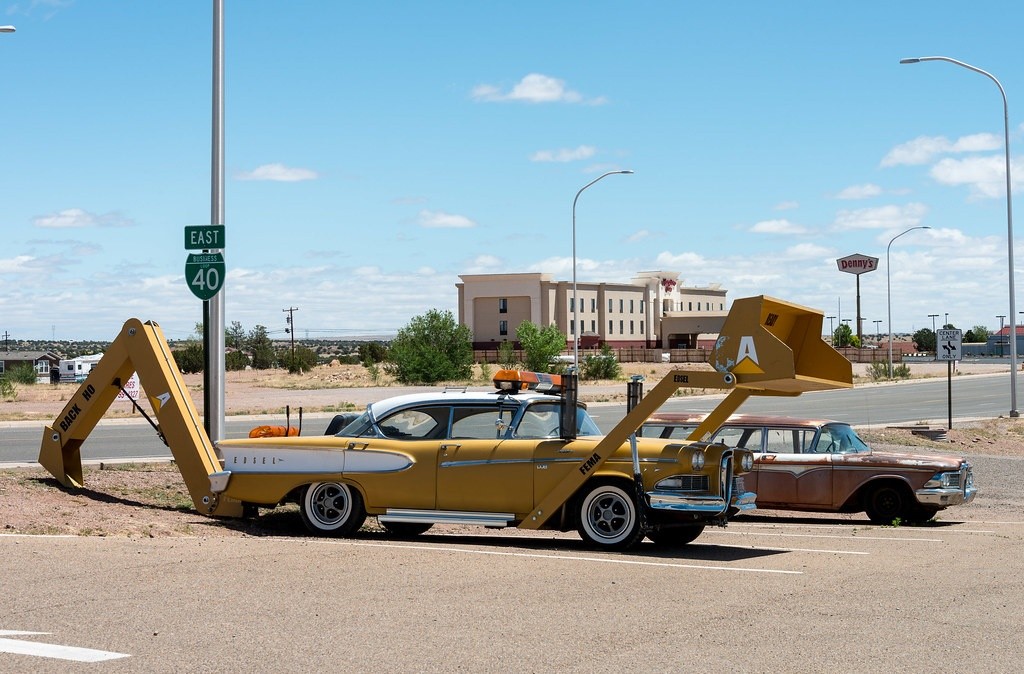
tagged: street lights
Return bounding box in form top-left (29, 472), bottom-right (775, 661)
top-left (572, 170), bottom-right (635, 380)
top-left (826, 316), bottom-right (836, 346)
top-left (860, 318), bottom-right (866, 349)
top-left (873, 321), bottom-right (882, 346)
top-left (842, 319), bottom-right (852, 327)
top-left (902, 56), bottom-right (1020, 420)
top-left (944, 314), bottom-right (948, 328)
top-left (887, 226), bottom-right (931, 379)
top-left (927, 314), bottom-right (939, 359)
top-left (995, 315), bottom-right (1006, 357)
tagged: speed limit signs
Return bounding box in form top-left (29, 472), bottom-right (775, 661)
top-left (184, 253), bottom-right (227, 301)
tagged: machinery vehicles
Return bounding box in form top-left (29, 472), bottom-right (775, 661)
top-left (37, 295), bottom-right (854, 550)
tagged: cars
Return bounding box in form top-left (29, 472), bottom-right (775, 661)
top-left (638, 414), bottom-right (976, 524)
